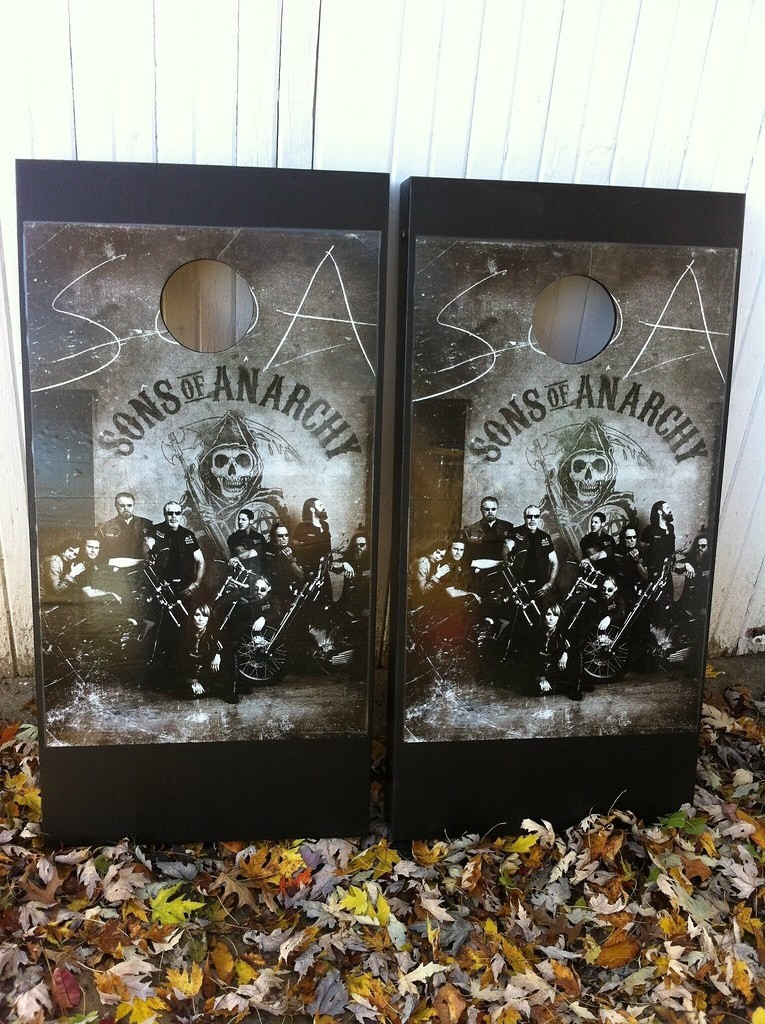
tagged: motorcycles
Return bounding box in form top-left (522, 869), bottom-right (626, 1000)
top-left (40, 528), bottom-right (370, 688)
top-left (407, 529), bottom-right (704, 682)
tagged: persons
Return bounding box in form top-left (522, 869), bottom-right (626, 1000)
top-left (39, 493), bottom-right (373, 705)
top-left (410, 497), bottom-right (711, 701)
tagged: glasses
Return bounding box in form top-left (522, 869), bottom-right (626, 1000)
top-left (625, 535), bottom-right (638, 540)
top-left (482, 507), bottom-right (497, 511)
top-left (353, 542), bottom-right (368, 549)
top-left (526, 514), bottom-right (540, 519)
top-left (601, 588), bottom-right (613, 593)
top-left (166, 511), bottom-right (182, 515)
top-left (116, 501), bottom-right (133, 509)
top-left (276, 533), bottom-right (290, 539)
top-left (254, 586), bottom-right (268, 593)
top-left (695, 542), bottom-right (708, 549)
